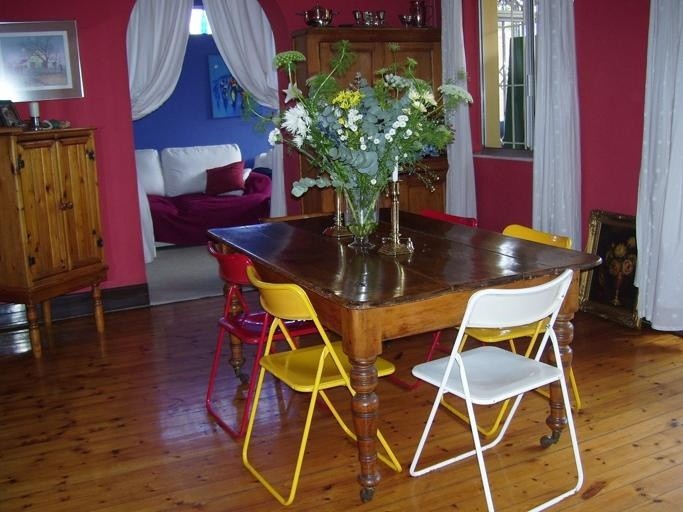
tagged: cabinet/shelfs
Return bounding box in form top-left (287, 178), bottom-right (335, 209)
top-left (290, 29), bottom-right (450, 215)
top-left (0, 126), bottom-right (110, 359)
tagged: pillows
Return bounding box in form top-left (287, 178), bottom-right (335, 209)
top-left (206, 160), bottom-right (246, 197)
top-left (217, 168), bottom-right (252, 196)
top-left (135, 149), bottom-right (164, 197)
top-left (161, 143), bottom-right (242, 197)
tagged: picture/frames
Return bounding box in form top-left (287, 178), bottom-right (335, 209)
top-left (578, 208), bottom-right (642, 329)
top-left (0, 19), bottom-right (85, 104)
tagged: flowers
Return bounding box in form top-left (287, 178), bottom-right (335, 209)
top-left (242, 38), bottom-right (474, 200)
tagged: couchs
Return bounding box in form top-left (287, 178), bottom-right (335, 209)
top-left (134, 143), bottom-right (271, 247)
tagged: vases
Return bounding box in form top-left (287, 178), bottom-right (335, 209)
top-left (343, 186), bottom-right (380, 251)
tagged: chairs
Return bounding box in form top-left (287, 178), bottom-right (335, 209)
top-left (386, 208), bottom-right (476, 391)
top-left (206, 240), bottom-right (326, 442)
top-left (240, 265), bottom-right (402, 506)
top-left (410, 269), bottom-right (584, 511)
top-left (442, 223), bottom-right (582, 434)
